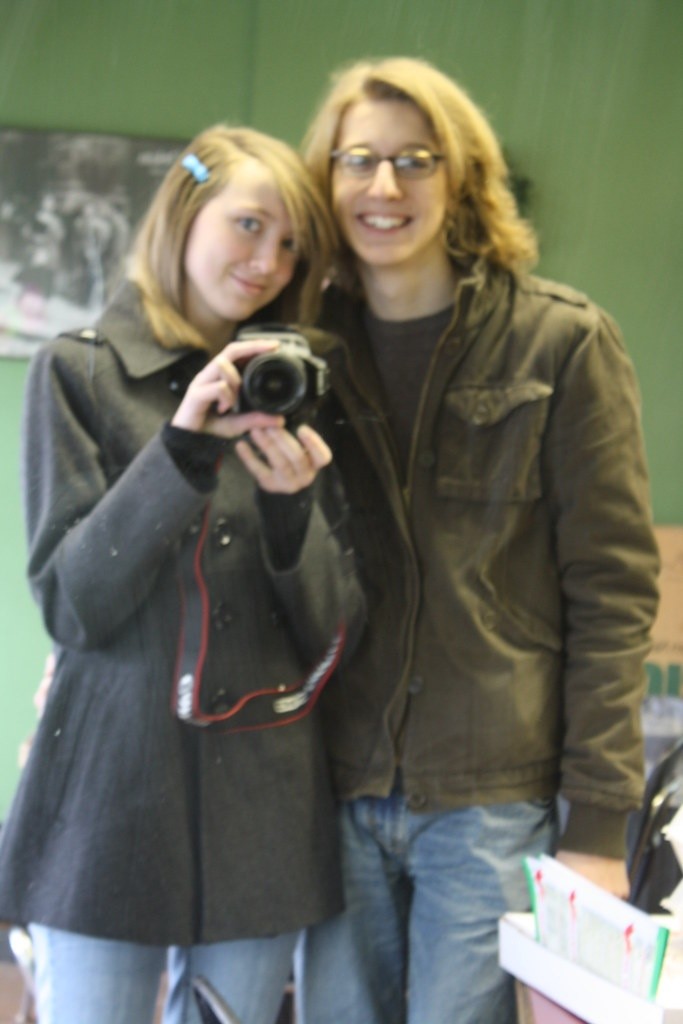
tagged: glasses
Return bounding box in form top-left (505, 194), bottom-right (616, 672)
top-left (330, 143), bottom-right (446, 180)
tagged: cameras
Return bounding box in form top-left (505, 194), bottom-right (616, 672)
top-left (234, 320), bottom-right (329, 430)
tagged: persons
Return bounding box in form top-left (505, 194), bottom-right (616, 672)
top-left (0, 124), bottom-right (370, 1024)
top-left (10, 193), bottom-right (127, 310)
top-left (293, 57), bottom-right (662, 1024)
